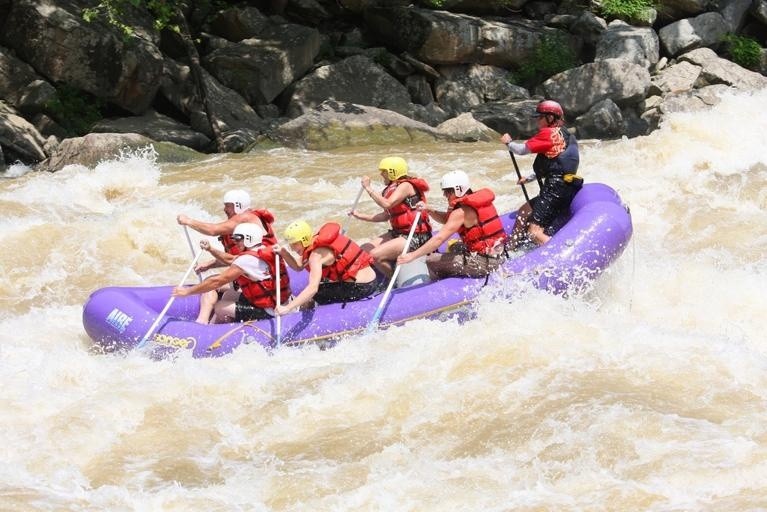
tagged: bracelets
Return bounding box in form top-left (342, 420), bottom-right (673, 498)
top-left (370, 189), bottom-right (375, 196)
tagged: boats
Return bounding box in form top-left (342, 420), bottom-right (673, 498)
top-left (81, 180), bottom-right (633, 361)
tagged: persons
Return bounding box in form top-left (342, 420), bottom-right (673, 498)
top-left (171, 223), bottom-right (290, 323)
top-left (177, 189), bottom-right (278, 324)
top-left (271, 219), bottom-right (378, 315)
top-left (347, 157), bottom-right (432, 288)
top-left (500, 100), bottom-right (584, 248)
top-left (397, 170), bottom-right (508, 281)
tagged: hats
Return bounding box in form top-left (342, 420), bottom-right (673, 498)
top-left (531, 112), bottom-right (543, 118)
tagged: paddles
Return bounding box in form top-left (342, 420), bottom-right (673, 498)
top-left (359, 205), bottom-right (425, 339)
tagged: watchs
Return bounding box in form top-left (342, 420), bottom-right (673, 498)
top-left (506, 139), bottom-right (513, 147)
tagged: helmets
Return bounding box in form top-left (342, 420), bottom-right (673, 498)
top-left (378, 156), bottom-right (409, 182)
top-left (440, 169), bottom-right (472, 197)
top-left (283, 220), bottom-right (314, 248)
top-left (536, 100), bottom-right (564, 121)
top-left (224, 189), bottom-right (251, 215)
top-left (232, 222), bottom-right (263, 248)
